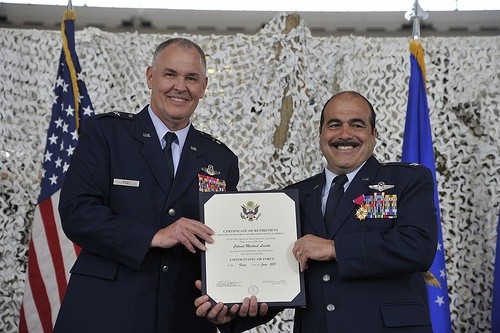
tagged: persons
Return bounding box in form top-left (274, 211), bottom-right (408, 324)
top-left (53, 37), bottom-right (269, 333)
top-left (193, 88), bottom-right (442, 333)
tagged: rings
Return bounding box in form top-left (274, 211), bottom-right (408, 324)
top-left (296, 251), bottom-right (302, 256)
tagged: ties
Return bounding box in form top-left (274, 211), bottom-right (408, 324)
top-left (324, 174), bottom-right (348, 233)
top-left (162, 132), bottom-right (177, 181)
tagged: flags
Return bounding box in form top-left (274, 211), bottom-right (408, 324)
top-left (16, 10), bottom-right (98, 333)
top-left (402, 42), bottom-right (453, 333)
